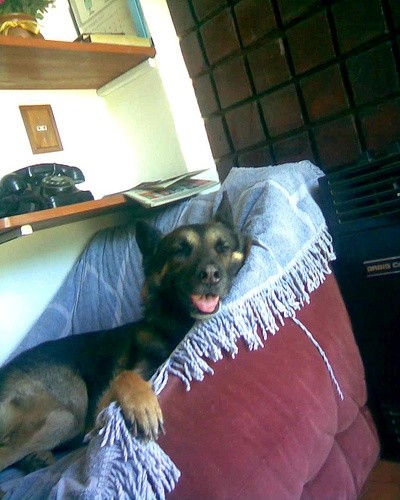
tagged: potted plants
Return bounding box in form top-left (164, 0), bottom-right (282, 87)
top-left (1, 0), bottom-right (56, 41)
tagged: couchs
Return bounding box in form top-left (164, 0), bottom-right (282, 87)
top-left (1, 159), bottom-right (381, 500)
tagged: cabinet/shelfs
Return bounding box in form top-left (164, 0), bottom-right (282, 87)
top-left (0, 0), bottom-right (224, 367)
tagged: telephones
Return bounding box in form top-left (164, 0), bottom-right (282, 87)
top-left (0, 163), bottom-right (95, 218)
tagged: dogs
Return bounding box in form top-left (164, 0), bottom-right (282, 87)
top-left (0, 191), bottom-right (252, 469)
